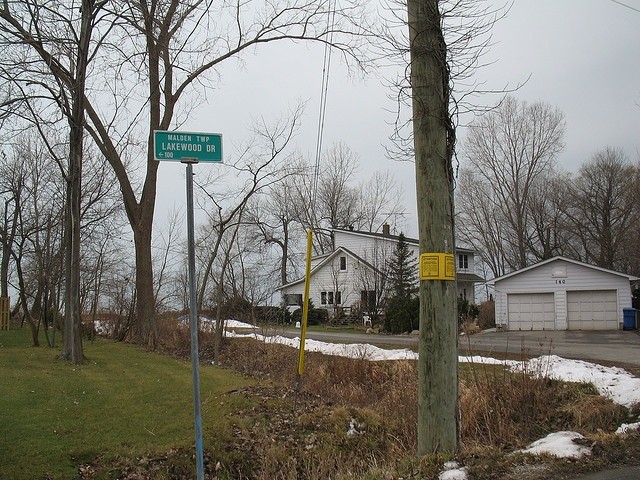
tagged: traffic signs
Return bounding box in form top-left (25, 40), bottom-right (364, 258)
top-left (153, 130), bottom-right (224, 164)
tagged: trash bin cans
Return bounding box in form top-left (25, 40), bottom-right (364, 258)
top-left (623, 308), bottom-right (637, 331)
